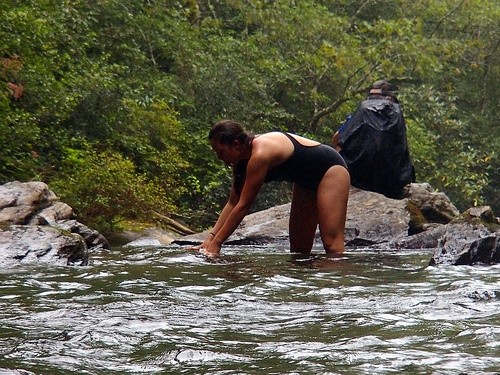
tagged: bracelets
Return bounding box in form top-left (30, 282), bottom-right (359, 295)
top-left (210, 232), bottom-right (215, 237)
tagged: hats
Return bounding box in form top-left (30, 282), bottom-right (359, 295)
top-left (367, 81), bottom-right (399, 104)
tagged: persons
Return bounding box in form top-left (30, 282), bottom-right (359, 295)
top-left (195, 120), bottom-right (350, 258)
